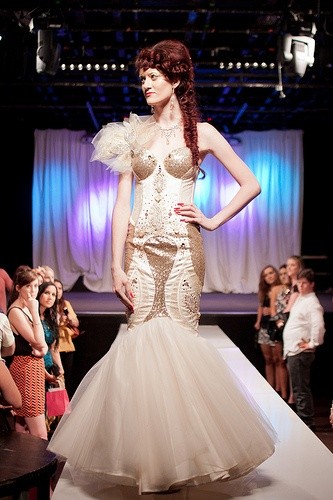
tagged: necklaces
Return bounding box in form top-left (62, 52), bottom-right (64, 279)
top-left (153, 115), bottom-right (184, 144)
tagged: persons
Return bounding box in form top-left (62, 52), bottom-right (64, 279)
top-left (254, 255), bottom-right (303, 406)
top-left (0, 313), bottom-right (21, 410)
top-left (44, 41), bottom-right (278, 495)
top-left (33, 265), bottom-right (78, 431)
top-left (283, 269), bottom-right (326, 431)
top-left (7, 266), bottom-right (47, 440)
top-left (36, 281), bottom-right (65, 430)
top-left (52, 280), bottom-right (80, 368)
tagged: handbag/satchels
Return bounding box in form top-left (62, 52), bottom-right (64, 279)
top-left (67, 325), bottom-right (80, 339)
top-left (46, 381), bottom-right (71, 417)
